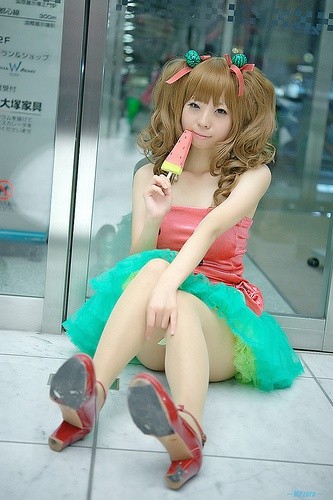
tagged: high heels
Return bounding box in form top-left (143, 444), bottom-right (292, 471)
top-left (48, 352), bottom-right (106, 451)
top-left (127, 372), bottom-right (208, 492)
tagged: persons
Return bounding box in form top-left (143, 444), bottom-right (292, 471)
top-left (47, 49), bottom-right (277, 492)
